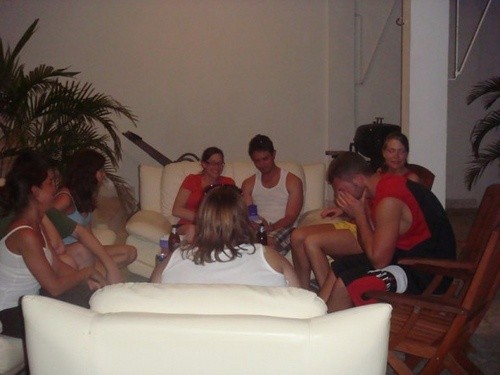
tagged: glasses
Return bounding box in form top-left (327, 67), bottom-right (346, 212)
top-left (205, 160), bottom-right (225, 168)
top-left (203, 184), bottom-right (245, 199)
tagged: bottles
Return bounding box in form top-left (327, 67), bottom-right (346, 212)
top-left (168, 224), bottom-right (180, 252)
top-left (257, 222), bottom-right (268, 246)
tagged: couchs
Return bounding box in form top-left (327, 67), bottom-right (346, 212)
top-left (126, 160), bottom-right (325, 279)
top-left (0, 176), bottom-right (116, 375)
top-left (20, 282), bottom-right (393, 375)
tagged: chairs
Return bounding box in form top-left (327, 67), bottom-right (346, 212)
top-left (363, 183), bottom-right (500, 375)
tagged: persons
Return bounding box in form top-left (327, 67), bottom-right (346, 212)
top-left (318, 153), bottom-right (457, 315)
top-left (240, 134), bottom-right (303, 257)
top-left (49, 148), bottom-right (137, 288)
top-left (172, 147), bottom-right (237, 246)
top-left (290, 132), bottom-right (425, 290)
top-left (148, 184), bottom-right (302, 289)
top-left (0, 154), bottom-right (126, 339)
